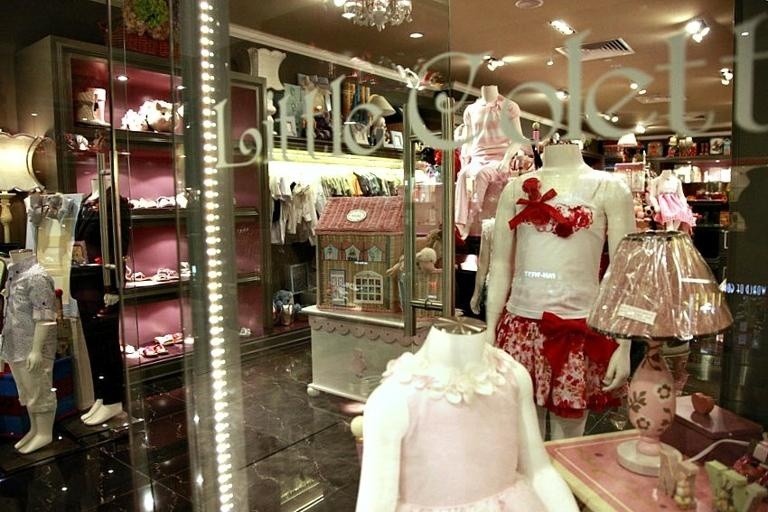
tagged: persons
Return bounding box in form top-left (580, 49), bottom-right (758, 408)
top-left (482, 140), bottom-right (638, 439)
top-left (274, 288), bottom-right (302, 315)
top-left (0, 238), bottom-right (58, 453)
top-left (453, 85), bottom-right (533, 240)
top-left (68, 170), bottom-right (133, 426)
top-left (649, 165), bottom-right (695, 234)
top-left (353, 316), bottom-right (579, 512)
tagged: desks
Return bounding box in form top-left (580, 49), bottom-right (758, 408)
top-left (542, 429), bottom-right (768, 512)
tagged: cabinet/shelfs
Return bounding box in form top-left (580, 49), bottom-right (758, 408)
top-left (271, 51), bottom-right (442, 329)
top-left (70, 57), bottom-right (264, 367)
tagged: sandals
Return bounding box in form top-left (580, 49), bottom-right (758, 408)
top-left (124, 260), bottom-right (192, 282)
top-left (126, 190), bottom-right (189, 209)
top-left (238, 328), bottom-right (252, 338)
top-left (119, 333), bottom-right (183, 359)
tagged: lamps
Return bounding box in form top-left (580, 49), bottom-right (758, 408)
top-left (586, 230), bottom-right (735, 478)
top-left (334, 0), bottom-right (413, 32)
top-left (0, 129), bottom-right (46, 243)
